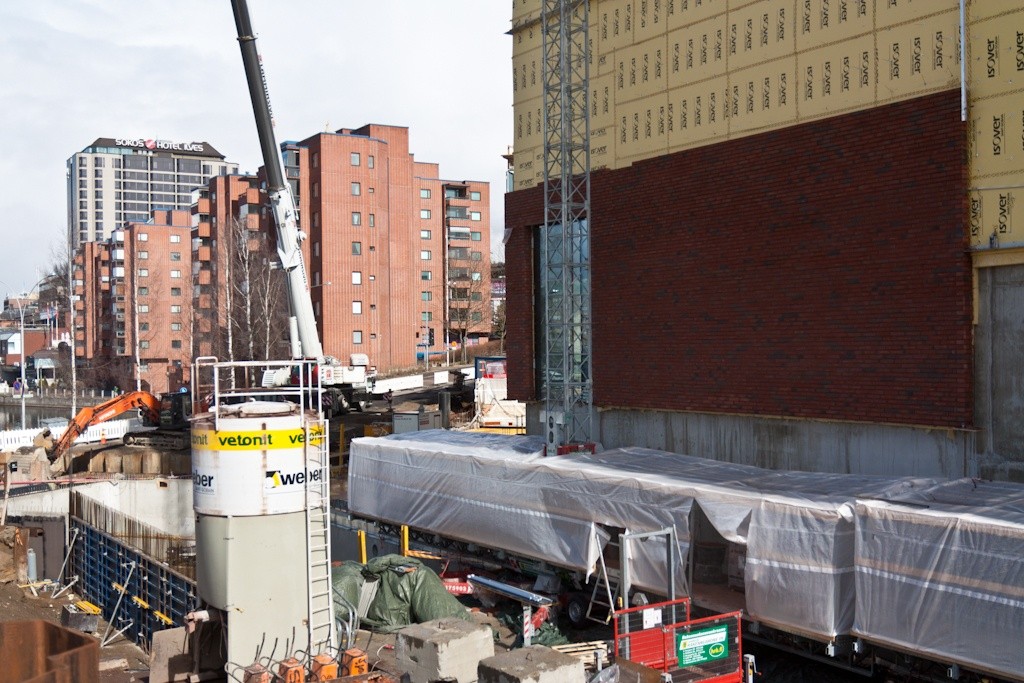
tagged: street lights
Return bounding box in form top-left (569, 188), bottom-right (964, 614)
top-left (1, 273), bottom-right (59, 430)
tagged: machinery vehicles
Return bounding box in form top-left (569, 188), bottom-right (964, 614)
top-left (37, 388), bottom-right (209, 463)
top-left (228, 0), bottom-right (396, 423)
